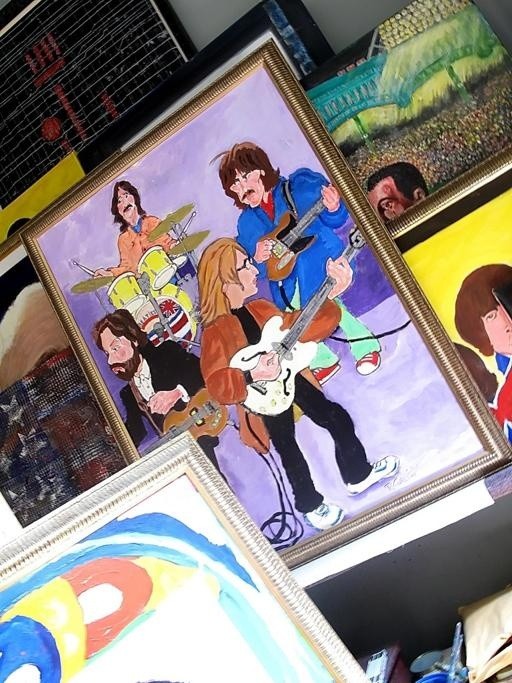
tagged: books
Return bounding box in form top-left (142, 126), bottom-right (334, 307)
top-left (459, 587), bottom-right (511, 683)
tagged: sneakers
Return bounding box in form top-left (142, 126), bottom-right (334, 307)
top-left (355, 350), bottom-right (383, 375)
top-left (313, 362), bottom-right (341, 387)
top-left (303, 502), bottom-right (346, 533)
top-left (343, 455), bottom-right (399, 495)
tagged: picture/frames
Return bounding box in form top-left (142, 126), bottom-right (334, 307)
top-left (0, 0), bottom-right (512, 683)
